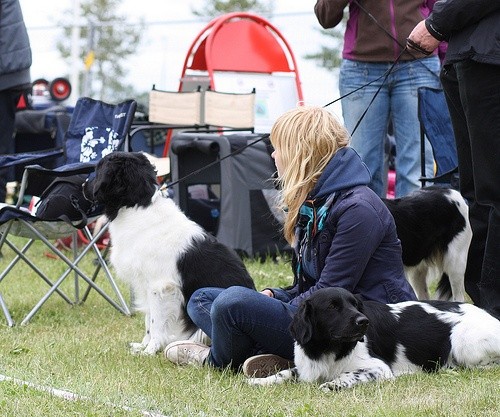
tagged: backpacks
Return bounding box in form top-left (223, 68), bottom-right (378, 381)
top-left (35, 174), bottom-right (92, 229)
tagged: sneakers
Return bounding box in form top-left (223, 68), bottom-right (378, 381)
top-left (164, 341), bottom-right (211, 368)
top-left (241, 354), bottom-right (293, 378)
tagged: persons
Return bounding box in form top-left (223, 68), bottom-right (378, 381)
top-left (405, 0), bottom-right (500, 321)
top-left (0, 0), bottom-right (32, 210)
top-left (164, 106), bottom-right (416, 380)
top-left (314, 0), bottom-right (443, 199)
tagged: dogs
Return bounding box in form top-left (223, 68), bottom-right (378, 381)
top-left (80, 152), bottom-right (258, 355)
top-left (380, 182), bottom-right (472, 302)
top-left (245, 285), bottom-right (500, 390)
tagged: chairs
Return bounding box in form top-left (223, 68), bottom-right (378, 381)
top-left (129, 83), bottom-right (256, 180)
top-left (0, 96), bottom-right (137, 326)
